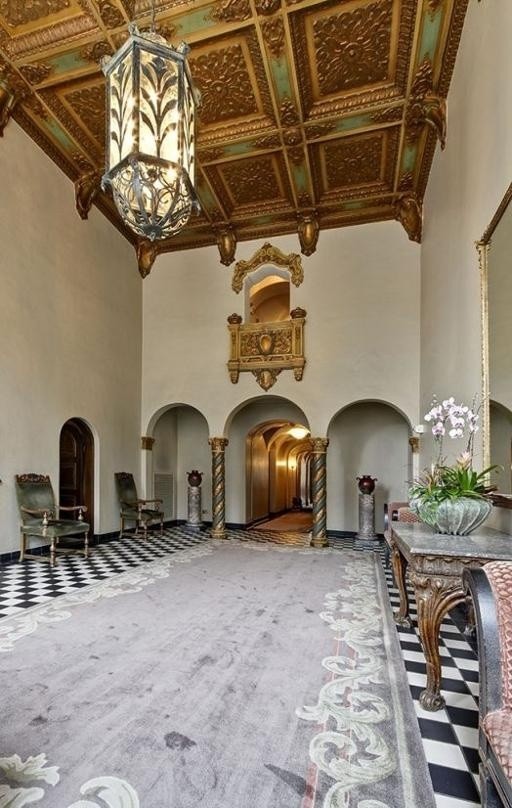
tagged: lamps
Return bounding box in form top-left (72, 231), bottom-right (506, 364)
top-left (283, 422), bottom-right (312, 441)
top-left (99, 0), bottom-right (207, 246)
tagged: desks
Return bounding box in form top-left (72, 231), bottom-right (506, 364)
top-left (385, 517), bottom-right (512, 714)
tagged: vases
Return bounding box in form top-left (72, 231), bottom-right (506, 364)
top-left (407, 485), bottom-right (494, 538)
top-left (355, 474), bottom-right (379, 496)
top-left (184, 469), bottom-right (205, 488)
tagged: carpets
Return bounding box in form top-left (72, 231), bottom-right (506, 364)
top-left (251, 511), bottom-right (319, 532)
top-left (0, 534), bottom-right (445, 808)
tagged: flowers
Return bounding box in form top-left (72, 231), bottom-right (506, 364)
top-left (401, 389), bottom-right (506, 531)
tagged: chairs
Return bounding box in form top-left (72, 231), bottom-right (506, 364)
top-left (460, 558), bottom-right (512, 808)
top-left (381, 499), bottom-right (424, 590)
top-left (114, 470), bottom-right (165, 542)
top-left (13, 473), bottom-right (91, 569)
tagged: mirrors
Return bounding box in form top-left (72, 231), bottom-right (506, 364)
top-left (472, 178), bottom-right (512, 512)
